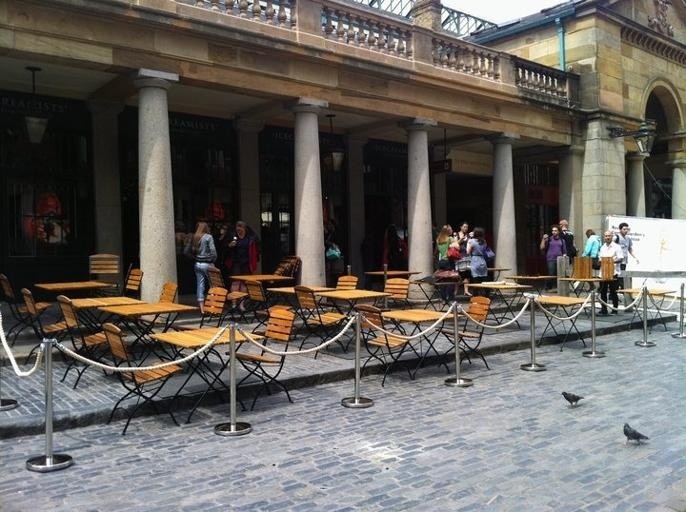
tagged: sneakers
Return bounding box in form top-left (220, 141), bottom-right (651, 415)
top-left (597, 310), bottom-right (609, 315)
top-left (609, 309), bottom-right (618, 316)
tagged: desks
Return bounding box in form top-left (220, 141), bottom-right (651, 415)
top-left (466, 268), bottom-right (677, 353)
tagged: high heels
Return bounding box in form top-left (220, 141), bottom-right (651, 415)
top-left (464, 292), bottom-right (473, 296)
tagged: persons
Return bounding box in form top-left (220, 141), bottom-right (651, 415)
top-left (323, 208), bottom-right (337, 287)
top-left (175, 218), bottom-right (260, 318)
top-left (433, 222), bottom-right (491, 298)
top-left (539, 218), bottom-right (641, 315)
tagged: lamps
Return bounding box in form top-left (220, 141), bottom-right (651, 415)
top-left (23, 67), bottom-right (48, 145)
top-left (324, 114), bottom-right (346, 172)
top-left (606, 119), bottom-right (658, 157)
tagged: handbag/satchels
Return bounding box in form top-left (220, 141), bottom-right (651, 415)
top-left (591, 257), bottom-right (601, 270)
top-left (484, 246), bottom-right (496, 258)
top-left (447, 246), bottom-right (463, 260)
